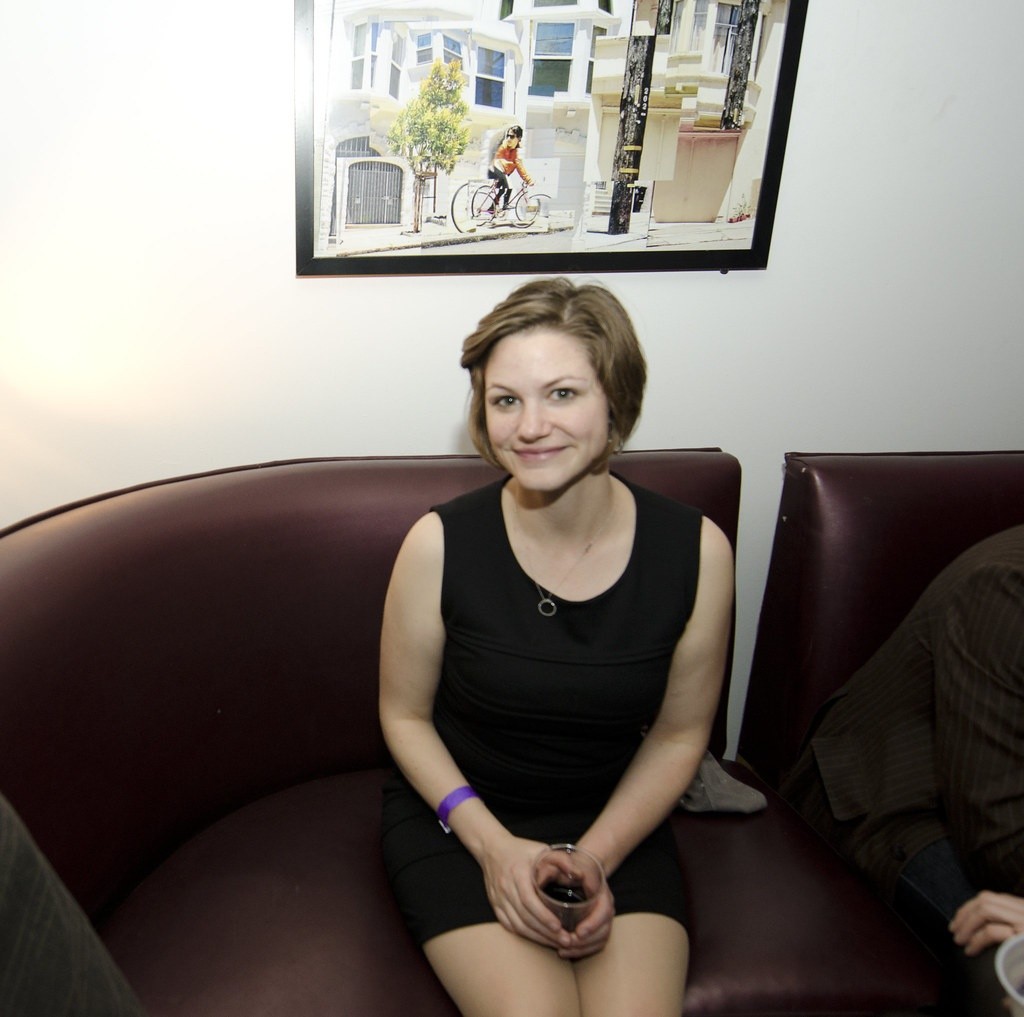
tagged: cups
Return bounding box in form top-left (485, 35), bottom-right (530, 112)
top-left (532, 844), bottom-right (604, 931)
top-left (995, 934), bottom-right (1024, 1017)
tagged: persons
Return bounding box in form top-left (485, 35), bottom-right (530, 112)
top-left (377, 278), bottom-right (733, 1017)
top-left (488, 125), bottom-right (534, 215)
top-left (949, 891), bottom-right (1024, 1017)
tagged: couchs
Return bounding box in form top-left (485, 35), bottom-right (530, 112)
top-left (0, 447), bottom-right (1024, 1017)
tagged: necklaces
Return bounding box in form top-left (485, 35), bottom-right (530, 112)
top-left (512, 488), bottom-right (616, 617)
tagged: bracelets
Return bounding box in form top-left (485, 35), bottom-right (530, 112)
top-left (436, 785), bottom-right (479, 834)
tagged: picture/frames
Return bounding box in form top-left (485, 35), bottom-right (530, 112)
top-left (293, 0), bottom-right (809, 278)
